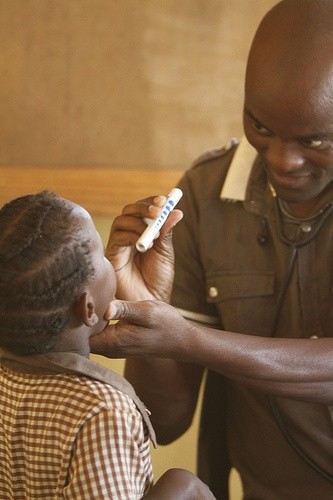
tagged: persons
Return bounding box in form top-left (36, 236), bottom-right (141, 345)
top-left (88, 0), bottom-right (332, 500)
top-left (0, 189), bottom-right (219, 500)
